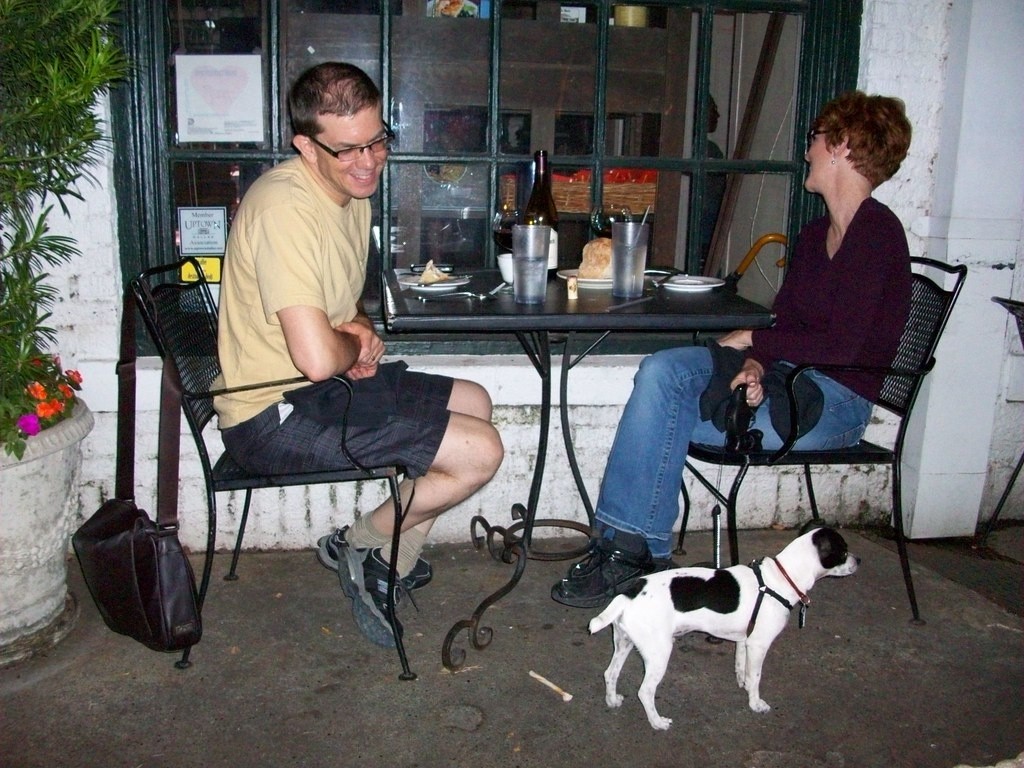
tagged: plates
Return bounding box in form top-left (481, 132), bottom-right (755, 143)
top-left (652, 276), bottom-right (726, 293)
top-left (396, 275), bottom-right (469, 291)
top-left (557, 269), bottom-right (612, 289)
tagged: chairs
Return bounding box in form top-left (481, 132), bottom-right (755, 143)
top-left (677, 255), bottom-right (968, 627)
top-left (986, 296), bottom-right (1023, 530)
top-left (132, 256), bottom-right (417, 682)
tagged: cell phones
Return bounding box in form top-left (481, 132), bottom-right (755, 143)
top-left (411, 263), bottom-right (454, 273)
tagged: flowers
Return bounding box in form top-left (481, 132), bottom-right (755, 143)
top-left (0, 1), bottom-right (150, 461)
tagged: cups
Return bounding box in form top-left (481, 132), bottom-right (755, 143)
top-left (512, 224), bottom-right (551, 305)
top-left (492, 211), bottom-right (522, 252)
top-left (590, 205), bottom-right (633, 240)
top-left (612, 223), bottom-right (649, 299)
top-left (497, 253), bottom-right (513, 285)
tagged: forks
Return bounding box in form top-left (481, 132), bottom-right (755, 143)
top-left (418, 282), bottom-right (506, 301)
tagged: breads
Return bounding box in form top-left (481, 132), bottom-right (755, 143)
top-left (578, 238), bottom-right (614, 280)
top-left (419, 258), bottom-right (450, 283)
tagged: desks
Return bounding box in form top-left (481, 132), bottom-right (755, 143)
top-left (381, 265), bottom-right (776, 671)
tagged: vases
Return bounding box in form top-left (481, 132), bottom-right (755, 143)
top-left (0, 398), bottom-right (94, 669)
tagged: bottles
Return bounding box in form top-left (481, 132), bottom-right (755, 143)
top-left (523, 150), bottom-right (559, 284)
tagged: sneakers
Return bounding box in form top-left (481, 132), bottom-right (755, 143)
top-left (339, 545), bottom-right (404, 648)
top-left (316, 523), bottom-right (434, 592)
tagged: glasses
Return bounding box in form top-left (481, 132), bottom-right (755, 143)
top-left (809, 124), bottom-right (840, 139)
top-left (310, 120), bottom-right (397, 163)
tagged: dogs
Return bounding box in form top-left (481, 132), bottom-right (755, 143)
top-left (588, 519), bottom-right (862, 732)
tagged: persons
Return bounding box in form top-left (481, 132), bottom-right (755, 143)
top-left (550, 91), bottom-right (911, 610)
top-left (690, 90), bottom-right (727, 276)
top-left (209, 61), bottom-right (505, 648)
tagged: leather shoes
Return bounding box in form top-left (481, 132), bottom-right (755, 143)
top-left (550, 544), bottom-right (680, 608)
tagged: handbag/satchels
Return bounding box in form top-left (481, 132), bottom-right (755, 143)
top-left (71, 496), bottom-right (204, 655)
